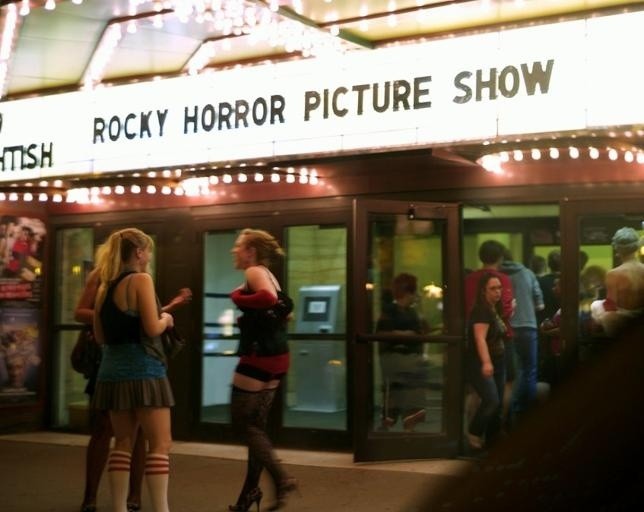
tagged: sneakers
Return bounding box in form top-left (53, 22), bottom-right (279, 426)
top-left (127, 499), bottom-right (140, 511)
top-left (81, 504), bottom-right (95, 512)
top-left (464, 428), bottom-right (481, 450)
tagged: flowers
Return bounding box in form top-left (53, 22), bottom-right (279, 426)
top-left (0, 323), bottom-right (41, 366)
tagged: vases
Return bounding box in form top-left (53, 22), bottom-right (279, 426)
top-left (2, 355), bottom-right (29, 393)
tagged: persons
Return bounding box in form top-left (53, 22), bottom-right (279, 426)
top-left (540, 273), bottom-right (562, 391)
top-left (498, 245), bottom-right (545, 413)
top-left (73, 267), bottom-right (191, 512)
top-left (375, 272), bottom-right (428, 428)
top-left (538, 250), bottom-right (560, 321)
top-left (463, 240), bottom-right (516, 428)
top-left (227, 227), bottom-right (304, 511)
top-left (590, 226), bottom-right (643, 331)
top-left (463, 274), bottom-right (508, 450)
top-left (93, 228), bottom-right (176, 512)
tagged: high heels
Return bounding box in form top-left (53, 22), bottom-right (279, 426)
top-left (265, 472), bottom-right (303, 512)
top-left (228, 487), bottom-right (262, 512)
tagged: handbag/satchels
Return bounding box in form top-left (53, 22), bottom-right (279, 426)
top-left (237, 267), bottom-right (294, 325)
